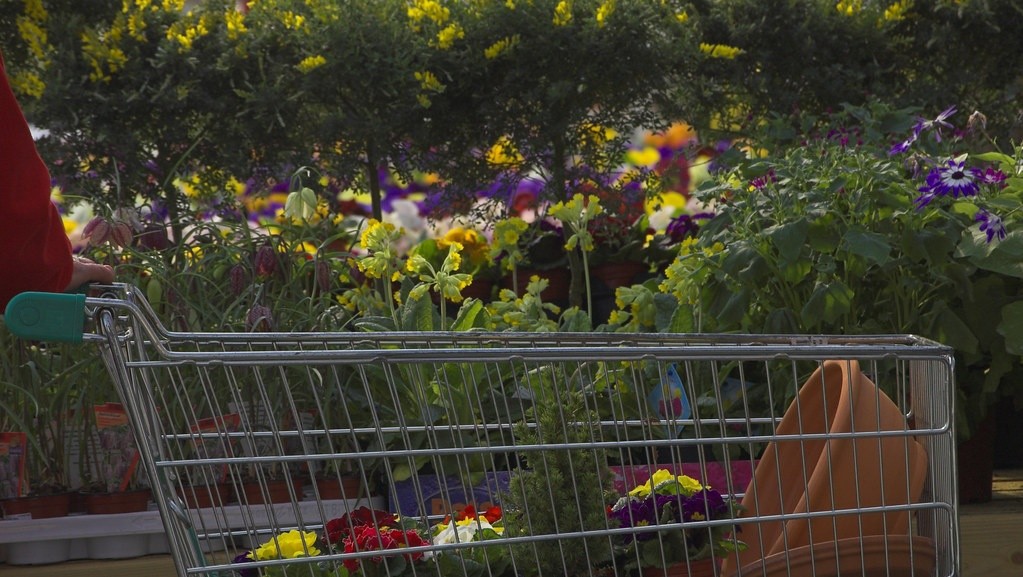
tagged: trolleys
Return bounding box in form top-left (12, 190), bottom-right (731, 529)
top-left (1, 248), bottom-right (960, 577)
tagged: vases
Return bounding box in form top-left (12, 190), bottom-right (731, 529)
top-left (730, 535), bottom-right (936, 577)
top-left (721, 360), bottom-right (927, 577)
top-left (641, 559), bottom-right (719, 577)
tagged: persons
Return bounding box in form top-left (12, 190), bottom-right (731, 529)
top-left (0, 59), bottom-right (120, 315)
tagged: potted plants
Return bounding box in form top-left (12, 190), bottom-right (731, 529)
top-left (76, 343), bottom-right (152, 514)
top-left (24, 340), bottom-right (77, 518)
top-left (1, 352), bottom-right (33, 515)
top-left (165, 295), bottom-right (361, 509)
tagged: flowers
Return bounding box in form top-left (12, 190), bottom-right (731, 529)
top-left (233, 469), bottom-right (749, 577)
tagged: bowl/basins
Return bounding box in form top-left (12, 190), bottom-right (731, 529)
top-left (720, 357), bottom-right (935, 577)
